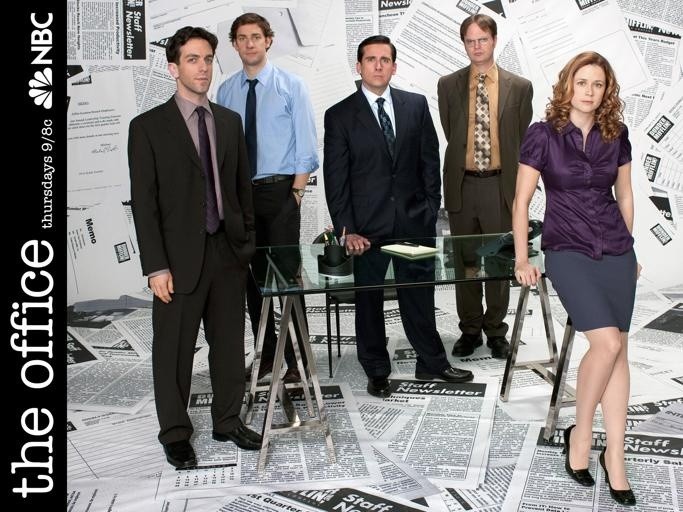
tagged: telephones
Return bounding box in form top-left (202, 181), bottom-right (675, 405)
top-left (501, 220), bottom-right (544, 245)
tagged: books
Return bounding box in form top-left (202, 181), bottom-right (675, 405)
top-left (380, 241), bottom-right (439, 261)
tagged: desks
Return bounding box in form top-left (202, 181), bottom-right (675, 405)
top-left (246, 233), bottom-right (576, 476)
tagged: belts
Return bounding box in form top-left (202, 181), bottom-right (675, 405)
top-left (250, 174), bottom-right (292, 186)
top-left (464, 169), bottom-right (501, 178)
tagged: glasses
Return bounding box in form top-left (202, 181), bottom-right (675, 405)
top-left (464, 37), bottom-right (487, 47)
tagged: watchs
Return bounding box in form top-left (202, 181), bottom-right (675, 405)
top-left (289, 187), bottom-right (304, 197)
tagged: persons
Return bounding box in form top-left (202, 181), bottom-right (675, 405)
top-left (506, 50), bottom-right (643, 506)
top-left (435, 13), bottom-right (536, 359)
top-left (211, 13), bottom-right (319, 388)
top-left (126, 26), bottom-right (273, 468)
top-left (321, 34), bottom-right (474, 397)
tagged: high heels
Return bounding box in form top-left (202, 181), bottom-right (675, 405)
top-left (562, 424), bottom-right (595, 486)
top-left (598, 446), bottom-right (636, 505)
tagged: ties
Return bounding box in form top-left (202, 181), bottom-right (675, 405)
top-left (194, 106), bottom-right (220, 235)
top-left (375, 97), bottom-right (395, 163)
top-left (244, 79), bottom-right (258, 179)
top-left (473, 74), bottom-right (491, 174)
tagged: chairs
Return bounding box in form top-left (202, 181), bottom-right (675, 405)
top-left (312, 226), bottom-right (400, 376)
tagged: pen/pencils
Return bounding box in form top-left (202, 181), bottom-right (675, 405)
top-left (395, 242), bottom-right (418, 247)
top-left (324, 226), bottom-right (346, 247)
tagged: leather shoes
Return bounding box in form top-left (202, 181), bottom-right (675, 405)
top-left (452, 328), bottom-right (482, 357)
top-left (163, 440), bottom-right (196, 468)
top-left (415, 361), bottom-right (473, 382)
top-left (245, 351), bottom-right (274, 381)
top-left (366, 375), bottom-right (390, 398)
top-left (283, 368), bottom-right (300, 383)
top-left (486, 336), bottom-right (510, 358)
top-left (212, 424), bottom-right (261, 450)
